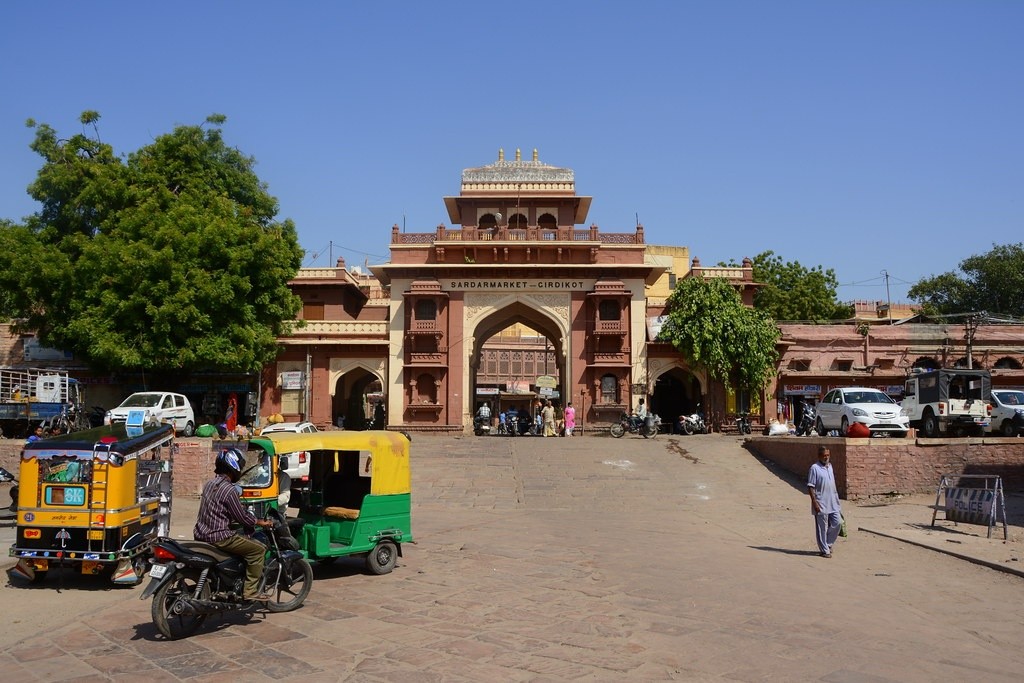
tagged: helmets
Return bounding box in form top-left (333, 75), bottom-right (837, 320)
top-left (213, 449), bottom-right (245, 482)
top-left (483, 403), bottom-right (487, 407)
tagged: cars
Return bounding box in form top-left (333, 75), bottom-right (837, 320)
top-left (794, 400), bottom-right (816, 436)
top-left (815, 387), bottom-right (910, 437)
top-left (977, 389), bottom-right (1024, 437)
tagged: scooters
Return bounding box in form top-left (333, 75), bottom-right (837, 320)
top-left (50, 403), bottom-right (90, 436)
top-left (474, 416), bottom-right (576, 437)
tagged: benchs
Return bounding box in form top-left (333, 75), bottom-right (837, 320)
top-left (320, 476), bottom-right (371, 544)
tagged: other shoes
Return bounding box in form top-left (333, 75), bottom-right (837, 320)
top-left (829, 547), bottom-right (833, 553)
top-left (821, 552), bottom-right (831, 558)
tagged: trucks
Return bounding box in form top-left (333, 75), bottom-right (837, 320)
top-left (0, 371), bottom-right (91, 438)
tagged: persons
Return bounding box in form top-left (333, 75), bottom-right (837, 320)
top-left (497, 410), bottom-right (505, 435)
top-left (257, 452), bottom-right (291, 518)
top-left (540, 401), bottom-right (557, 438)
top-left (472, 402), bottom-right (491, 431)
top-left (506, 403), bottom-right (520, 432)
top-left (49, 424), bottom-right (60, 436)
top-left (807, 446), bottom-right (843, 558)
top-left (564, 402), bottom-right (575, 437)
top-left (536, 412), bottom-right (541, 434)
top-left (629, 398), bottom-right (646, 432)
top-left (374, 400), bottom-right (386, 429)
top-left (193, 447), bottom-right (272, 601)
top-left (26, 426), bottom-right (44, 444)
top-left (554, 402), bottom-right (565, 437)
top-left (14, 388), bottom-right (21, 402)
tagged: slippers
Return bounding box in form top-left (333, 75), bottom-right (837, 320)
top-left (245, 591), bottom-right (270, 602)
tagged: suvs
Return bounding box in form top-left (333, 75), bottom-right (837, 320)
top-left (258, 421), bottom-right (318, 435)
top-left (896, 370), bottom-right (992, 438)
top-left (104, 392), bottom-right (196, 437)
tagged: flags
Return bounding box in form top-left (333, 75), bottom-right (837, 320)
top-left (225, 393), bottom-right (237, 432)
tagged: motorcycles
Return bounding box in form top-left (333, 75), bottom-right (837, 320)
top-left (8, 410), bottom-right (180, 586)
top-left (234, 431), bottom-right (418, 576)
top-left (678, 403), bottom-right (708, 435)
top-left (735, 412), bottom-right (752, 435)
top-left (140, 485), bottom-right (313, 639)
top-left (610, 409), bottom-right (663, 439)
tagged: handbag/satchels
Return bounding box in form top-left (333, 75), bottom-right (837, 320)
top-left (839, 512), bottom-right (847, 537)
